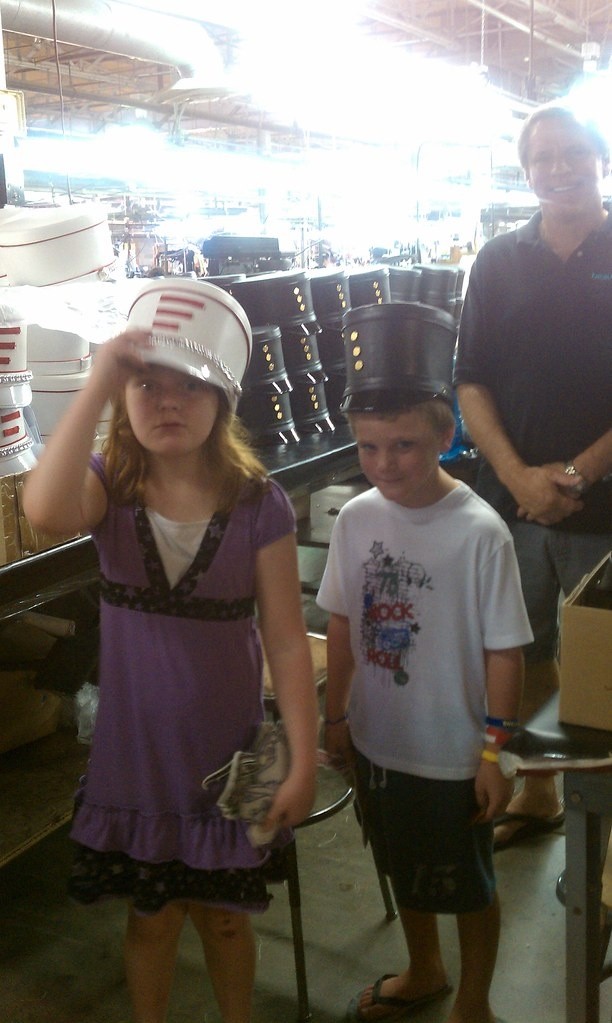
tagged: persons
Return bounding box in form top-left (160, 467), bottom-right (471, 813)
top-left (22, 278), bottom-right (319, 1023)
top-left (455, 106), bottom-right (612, 853)
top-left (314, 302), bottom-right (534, 1023)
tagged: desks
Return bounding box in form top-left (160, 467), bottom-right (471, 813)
top-left (0, 422), bottom-right (365, 622)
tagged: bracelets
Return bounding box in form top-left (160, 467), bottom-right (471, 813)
top-left (326, 717), bottom-right (347, 725)
top-left (481, 750), bottom-right (499, 763)
top-left (484, 727), bottom-right (514, 745)
top-left (486, 717), bottom-right (518, 727)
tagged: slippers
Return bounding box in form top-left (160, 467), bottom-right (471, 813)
top-left (345, 973), bottom-right (454, 1023)
top-left (491, 806), bottom-right (567, 853)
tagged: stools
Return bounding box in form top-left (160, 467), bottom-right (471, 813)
top-left (261, 632), bottom-right (399, 1023)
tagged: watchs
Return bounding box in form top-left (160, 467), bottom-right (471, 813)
top-left (565, 459), bottom-right (585, 494)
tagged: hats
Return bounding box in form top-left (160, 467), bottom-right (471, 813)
top-left (0, 263), bottom-right (466, 483)
